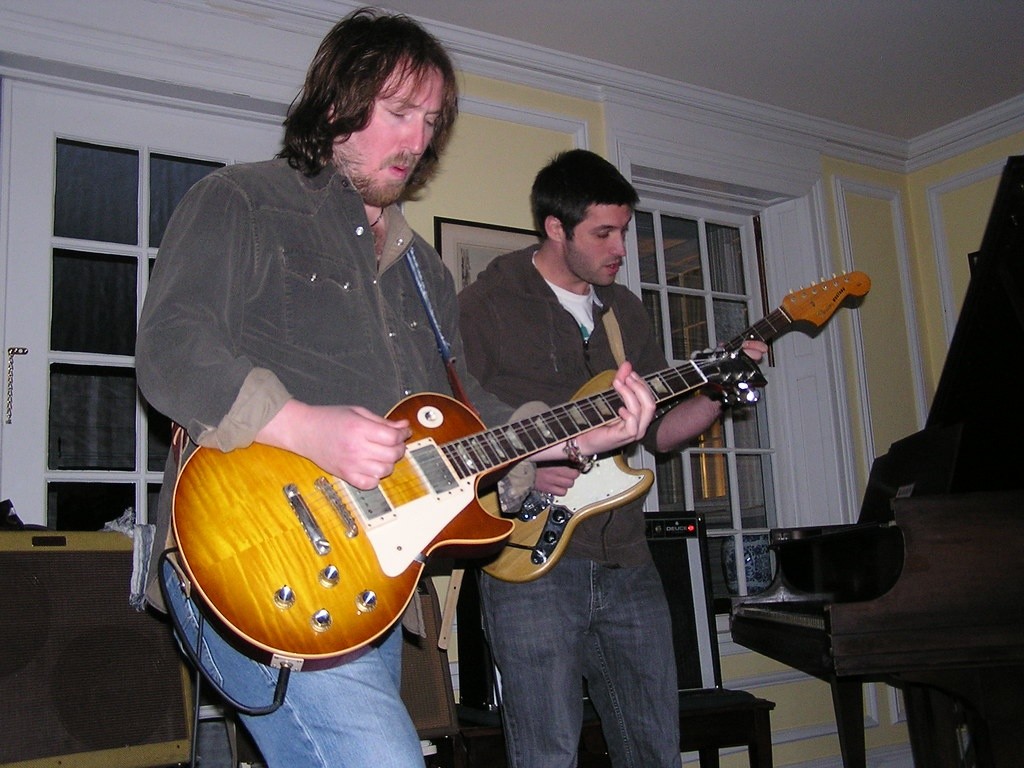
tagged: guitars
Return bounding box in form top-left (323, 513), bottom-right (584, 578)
top-left (170, 342), bottom-right (770, 673)
top-left (479, 268), bottom-right (872, 584)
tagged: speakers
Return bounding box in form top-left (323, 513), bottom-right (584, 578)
top-left (0, 529), bottom-right (195, 768)
top-left (643, 510), bottom-right (723, 692)
top-left (400, 579), bottom-right (458, 739)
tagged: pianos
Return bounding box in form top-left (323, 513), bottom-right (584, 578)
top-left (729, 153), bottom-right (1023, 768)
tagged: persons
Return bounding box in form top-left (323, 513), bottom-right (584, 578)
top-left (459, 150), bottom-right (768, 768)
top-left (136, 2), bottom-right (657, 768)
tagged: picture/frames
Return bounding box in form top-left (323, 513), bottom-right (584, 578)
top-left (433, 216), bottom-right (542, 294)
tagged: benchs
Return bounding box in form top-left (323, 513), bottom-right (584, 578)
top-left (463, 688), bottom-right (777, 768)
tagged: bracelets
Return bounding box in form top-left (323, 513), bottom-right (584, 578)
top-left (563, 438), bottom-right (588, 465)
